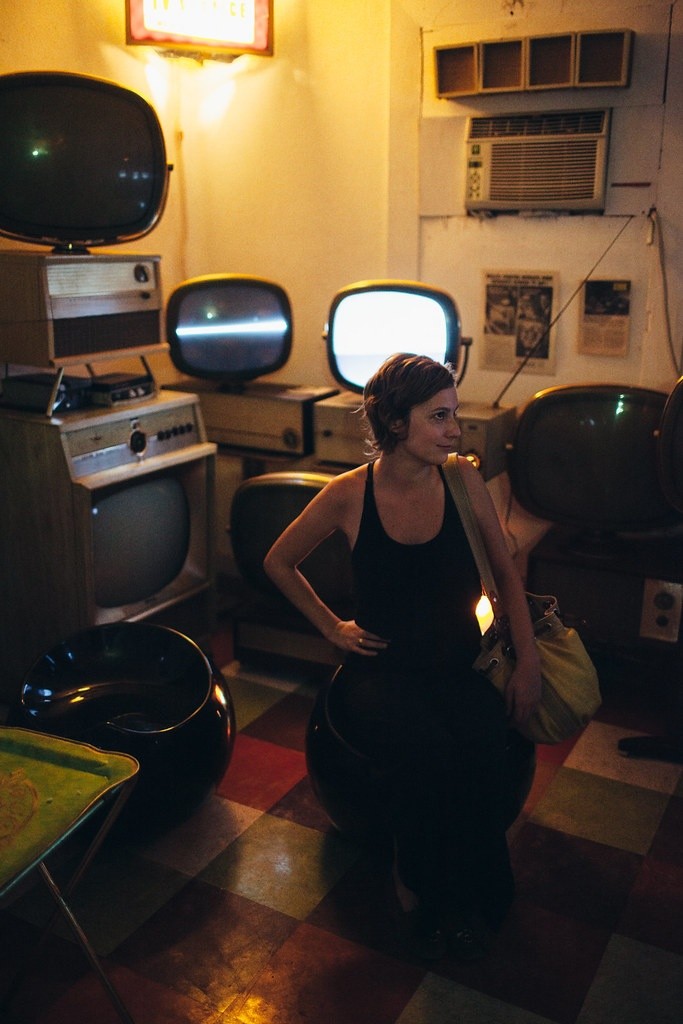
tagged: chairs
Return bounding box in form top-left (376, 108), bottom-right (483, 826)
top-left (0, 725), bottom-right (142, 1024)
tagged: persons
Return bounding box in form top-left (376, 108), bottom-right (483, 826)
top-left (261, 352), bottom-right (541, 907)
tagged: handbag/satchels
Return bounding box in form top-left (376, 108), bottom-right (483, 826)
top-left (472, 590), bottom-right (603, 745)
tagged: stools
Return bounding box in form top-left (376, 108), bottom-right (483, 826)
top-left (298, 652), bottom-right (535, 845)
top-left (7, 620), bottom-right (234, 836)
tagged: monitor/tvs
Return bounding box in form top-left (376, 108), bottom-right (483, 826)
top-left (506, 383), bottom-right (683, 531)
top-left (72, 442), bottom-right (218, 625)
top-left (229, 471), bottom-right (352, 604)
top-left (0, 69), bottom-right (169, 254)
top-left (165, 273), bottom-right (294, 392)
top-left (325, 279), bottom-right (462, 396)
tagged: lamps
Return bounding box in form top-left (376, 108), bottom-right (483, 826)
top-left (124, 0), bottom-right (274, 70)
